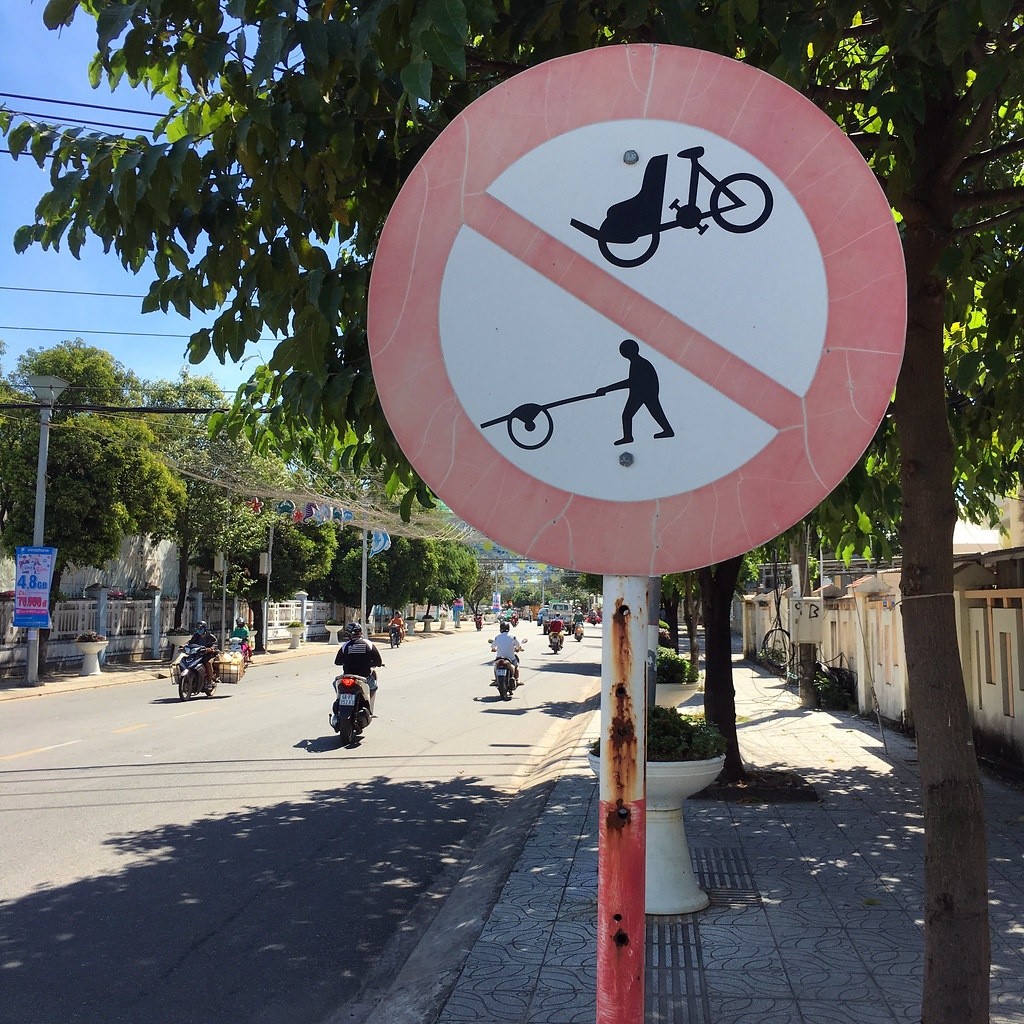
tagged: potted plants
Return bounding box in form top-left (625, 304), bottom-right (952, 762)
top-left (654, 644), bottom-right (700, 713)
top-left (165, 628), bottom-right (193, 663)
top-left (585, 703), bottom-right (728, 914)
top-left (75, 630), bottom-right (109, 675)
top-left (404, 613), bottom-right (448, 634)
top-left (244, 617), bottom-right (344, 649)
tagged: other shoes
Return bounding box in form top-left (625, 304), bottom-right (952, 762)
top-left (204, 683), bottom-right (218, 687)
top-left (516, 681), bottom-right (525, 685)
top-left (490, 681), bottom-right (498, 686)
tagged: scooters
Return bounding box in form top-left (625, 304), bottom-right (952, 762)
top-left (329, 663), bottom-right (386, 744)
top-left (224, 636), bottom-right (250, 669)
top-left (177, 645), bottom-right (220, 701)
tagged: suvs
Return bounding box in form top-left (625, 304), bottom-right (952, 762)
top-left (537, 605), bottom-right (550, 625)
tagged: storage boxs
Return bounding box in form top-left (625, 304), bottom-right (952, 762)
top-left (172, 653), bottom-right (189, 683)
top-left (218, 655), bottom-right (244, 683)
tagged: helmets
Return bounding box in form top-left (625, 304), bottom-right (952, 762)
top-left (345, 621), bottom-right (363, 641)
top-left (236, 617), bottom-right (245, 627)
top-left (194, 620), bottom-right (208, 635)
top-left (499, 620), bottom-right (510, 632)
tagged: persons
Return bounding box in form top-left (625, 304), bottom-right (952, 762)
top-left (573, 607), bottom-right (585, 625)
top-left (335, 622), bottom-right (382, 699)
top-left (589, 608), bottom-right (602, 619)
top-left (549, 612), bottom-right (564, 644)
top-left (491, 621), bottom-right (522, 679)
top-left (187, 621), bottom-right (218, 685)
top-left (388, 611), bottom-right (405, 642)
top-left (230, 618), bottom-right (252, 662)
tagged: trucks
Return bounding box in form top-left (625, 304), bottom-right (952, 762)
top-left (542, 602), bottom-right (575, 634)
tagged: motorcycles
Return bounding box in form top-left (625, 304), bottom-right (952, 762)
top-left (509, 616), bottom-right (519, 627)
top-left (474, 615), bottom-right (484, 631)
top-left (572, 619), bottom-right (585, 641)
top-left (589, 615), bottom-right (597, 626)
top-left (390, 623), bottom-right (402, 648)
top-left (490, 637), bottom-right (528, 700)
top-left (546, 625), bottom-right (565, 654)
top-left (499, 613), bottom-right (506, 624)
